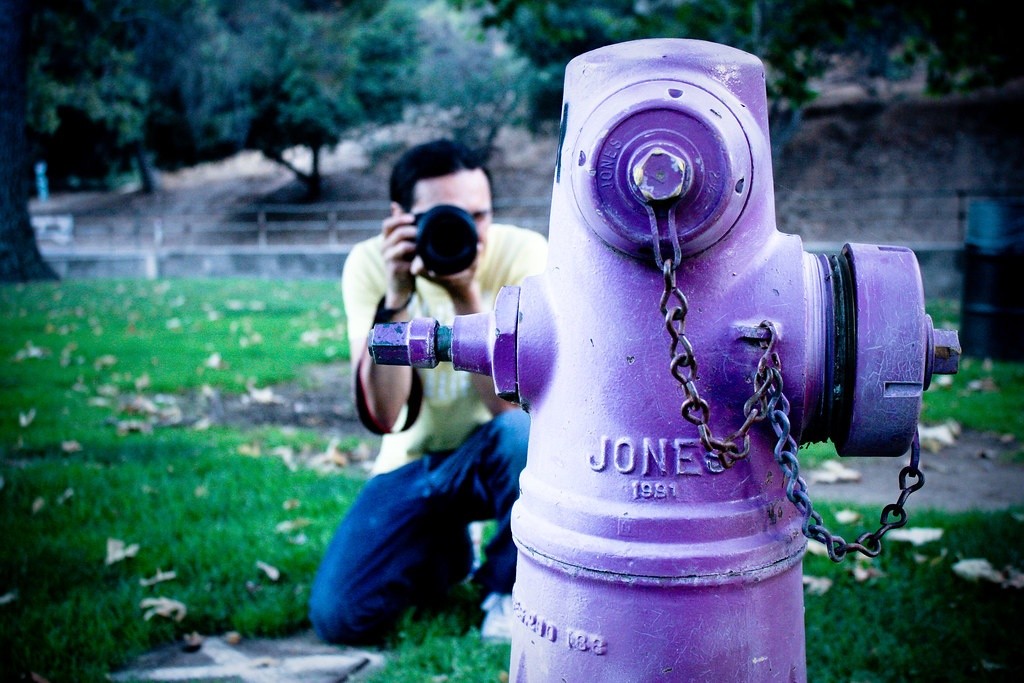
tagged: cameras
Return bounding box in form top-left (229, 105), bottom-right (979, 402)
top-left (399, 204), bottom-right (480, 276)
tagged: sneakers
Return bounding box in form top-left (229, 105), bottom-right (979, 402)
top-left (481, 593), bottom-right (513, 639)
top-left (466, 521), bottom-right (484, 581)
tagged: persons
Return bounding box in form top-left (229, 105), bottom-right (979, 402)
top-left (308, 140), bottom-right (548, 648)
